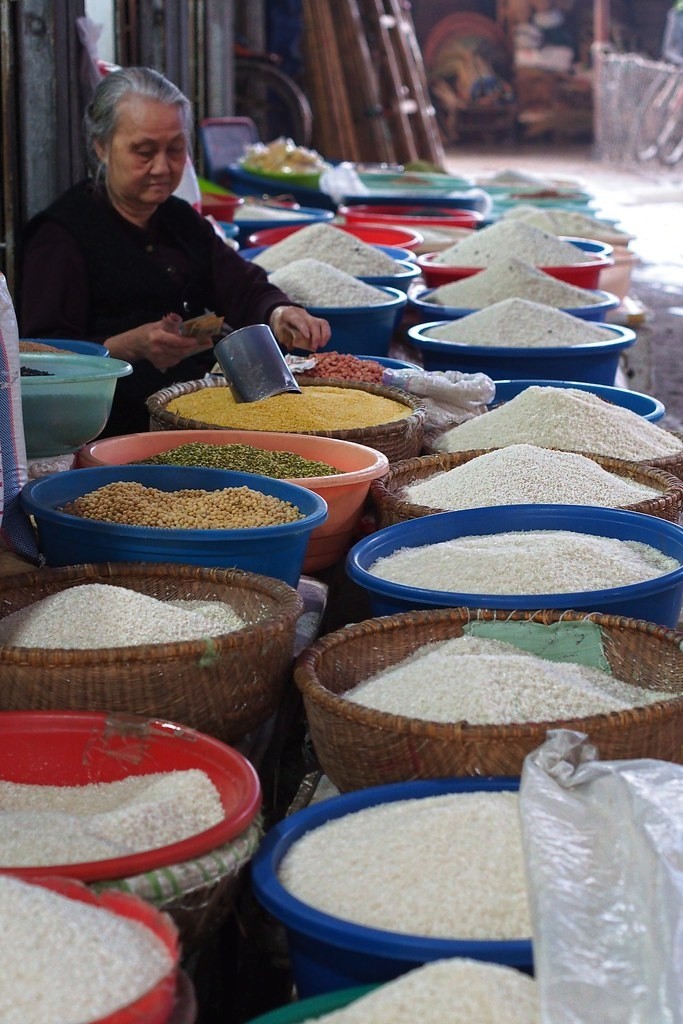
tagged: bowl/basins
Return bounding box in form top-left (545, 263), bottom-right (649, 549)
top-left (347, 504), bottom-right (683, 631)
top-left (251, 777), bottom-right (535, 999)
top-left (24, 877), bottom-right (180, 1024)
top-left (82, 430), bottom-right (390, 571)
top-left (19, 466), bottom-right (328, 590)
top-left (198, 164), bottom-right (665, 423)
top-left (250, 984), bottom-right (382, 1024)
top-left (19, 352), bottom-right (133, 458)
top-left (0, 710), bottom-right (259, 888)
top-left (20, 339), bottom-right (109, 360)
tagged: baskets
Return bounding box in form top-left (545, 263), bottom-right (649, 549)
top-left (293, 606), bottom-right (683, 795)
top-left (85, 811), bottom-right (264, 969)
top-left (145, 375), bottom-right (683, 532)
top-left (0, 562), bottom-right (305, 743)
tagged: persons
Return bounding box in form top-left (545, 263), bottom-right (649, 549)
top-left (17, 65), bottom-right (330, 439)
